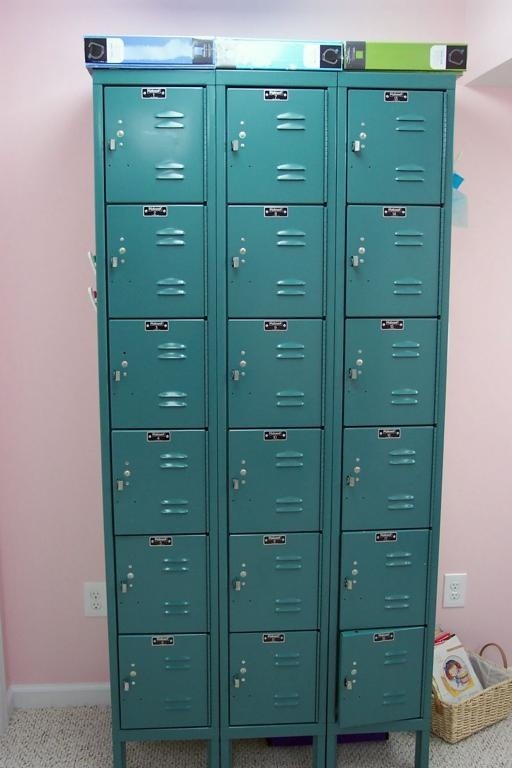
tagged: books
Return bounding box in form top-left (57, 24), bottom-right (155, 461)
top-left (431, 626), bottom-right (484, 705)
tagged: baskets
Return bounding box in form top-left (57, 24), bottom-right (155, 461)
top-left (432, 642), bottom-right (511, 744)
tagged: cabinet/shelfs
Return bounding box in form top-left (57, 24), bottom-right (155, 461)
top-left (86, 66), bottom-right (458, 768)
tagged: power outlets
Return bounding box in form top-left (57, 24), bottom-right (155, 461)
top-left (444, 575), bottom-right (468, 608)
top-left (86, 582), bottom-right (109, 619)
